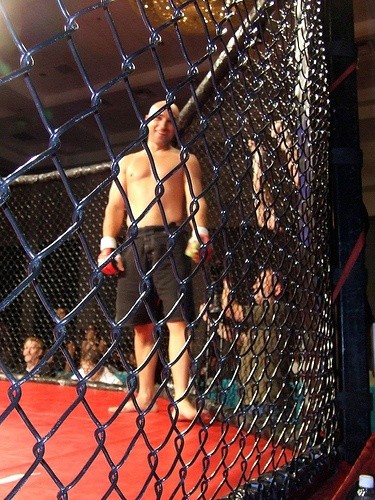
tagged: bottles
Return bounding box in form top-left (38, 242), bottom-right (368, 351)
top-left (354, 475), bottom-right (375, 500)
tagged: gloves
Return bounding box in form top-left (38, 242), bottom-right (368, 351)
top-left (97, 236), bottom-right (124, 275)
top-left (186, 225), bottom-right (213, 263)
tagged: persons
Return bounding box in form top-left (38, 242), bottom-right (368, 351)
top-left (20, 119), bottom-right (314, 429)
top-left (96, 101), bottom-right (213, 422)
